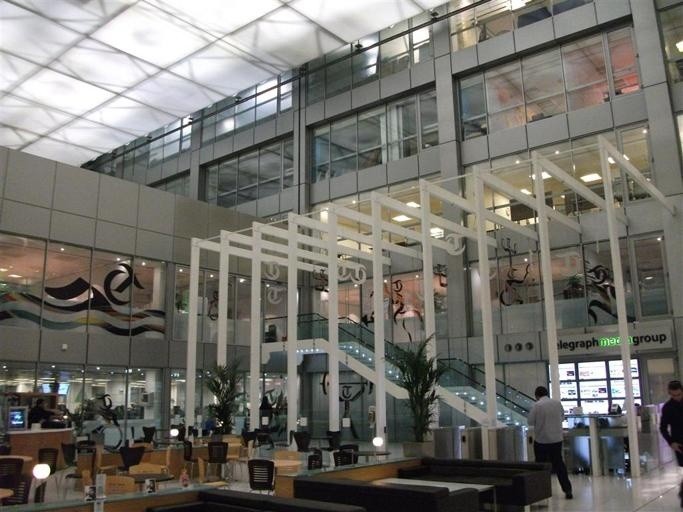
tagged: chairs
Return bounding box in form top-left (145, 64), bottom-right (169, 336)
top-left (1, 426), bottom-right (358, 506)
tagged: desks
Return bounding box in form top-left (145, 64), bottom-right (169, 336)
top-left (354, 451), bottom-right (391, 462)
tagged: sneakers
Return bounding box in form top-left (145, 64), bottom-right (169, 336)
top-left (566, 493), bottom-right (572, 499)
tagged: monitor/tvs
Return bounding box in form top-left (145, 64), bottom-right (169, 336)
top-left (7, 405), bottom-right (28, 431)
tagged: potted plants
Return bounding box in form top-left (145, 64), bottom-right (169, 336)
top-left (197, 355), bottom-right (248, 442)
top-left (380, 329), bottom-right (452, 458)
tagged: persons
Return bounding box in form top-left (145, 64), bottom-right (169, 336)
top-left (28, 399), bottom-right (60, 428)
top-left (259, 395), bottom-right (274, 450)
top-left (660, 381), bottom-right (683, 508)
top-left (527, 385), bottom-right (572, 498)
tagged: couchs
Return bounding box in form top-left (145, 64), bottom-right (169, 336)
top-left (293, 458), bottom-right (552, 512)
